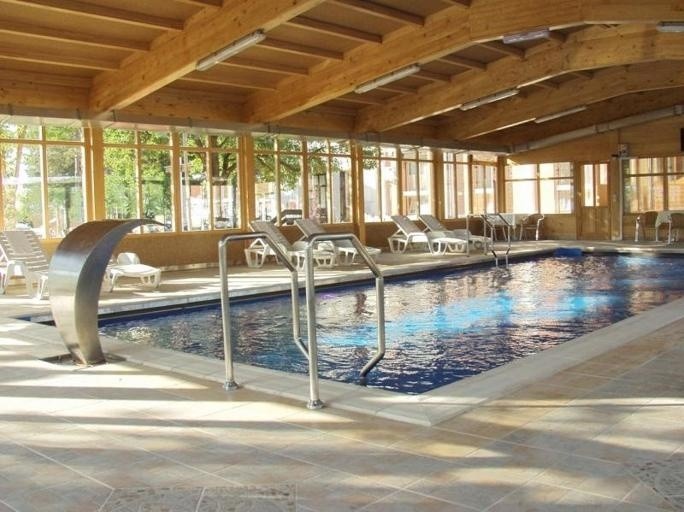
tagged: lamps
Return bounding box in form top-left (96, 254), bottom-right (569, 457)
top-left (501, 26), bottom-right (550, 46)
top-left (352, 62), bottom-right (420, 95)
top-left (458, 87), bottom-right (519, 112)
top-left (195, 26), bottom-right (266, 72)
top-left (533, 104), bottom-right (586, 123)
top-left (655, 21), bottom-right (684, 32)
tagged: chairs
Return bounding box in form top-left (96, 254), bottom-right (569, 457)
top-left (481, 215), bottom-right (511, 242)
top-left (1, 231), bottom-right (52, 300)
top-left (292, 218), bottom-right (381, 268)
top-left (416, 215), bottom-right (492, 253)
top-left (62, 225), bottom-right (164, 291)
top-left (241, 220), bottom-right (337, 269)
top-left (519, 212), bottom-right (545, 241)
top-left (633, 210), bottom-right (683, 246)
top-left (386, 216), bottom-right (467, 256)
